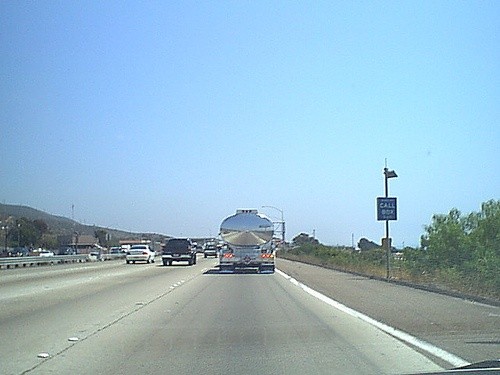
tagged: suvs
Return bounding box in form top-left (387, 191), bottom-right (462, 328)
top-left (161, 237), bottom-right (198, 265)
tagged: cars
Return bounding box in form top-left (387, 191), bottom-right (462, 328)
top-left (126, 244), bottom-right (157, 263)
top-left (4, 243), bottom-right (130, 262)
top-left (271, 238), bottom-right (284, 250)
top-left (191, 239), bottom-right (227, 259)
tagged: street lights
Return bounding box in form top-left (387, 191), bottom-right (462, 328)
top-left (383, 157), bottom-right (398, 279)
top-left (262, 205), bottom-right (285, 247)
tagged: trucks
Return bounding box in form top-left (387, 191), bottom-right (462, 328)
top-left (218, 209), bottom-right (278, 275)
